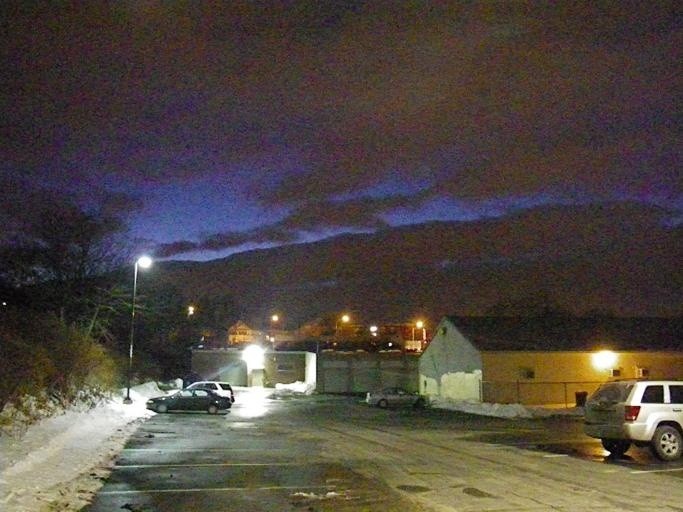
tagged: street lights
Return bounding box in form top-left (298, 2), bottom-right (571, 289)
top-left (368, 325), bottom-right (377, 348)
top-left (336, 315), bottom-right (349, 330)
top-left (412, 321), bottom-right (423, 342)
top-left (271, 315), bottom-right (278, 338)
top-left (125, 256), bottom-right (152, 399)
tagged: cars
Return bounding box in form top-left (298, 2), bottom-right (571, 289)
top-left (365, 387), bottom-right (429, 410)
top-left (145, 381), bottom-right (235, 414)
top-left (582, 377), bottom-right (683, 461)
top-left (332, 340), bottom-right (354, 351)
top-left (165, 365), bottom-right (202, 387)
